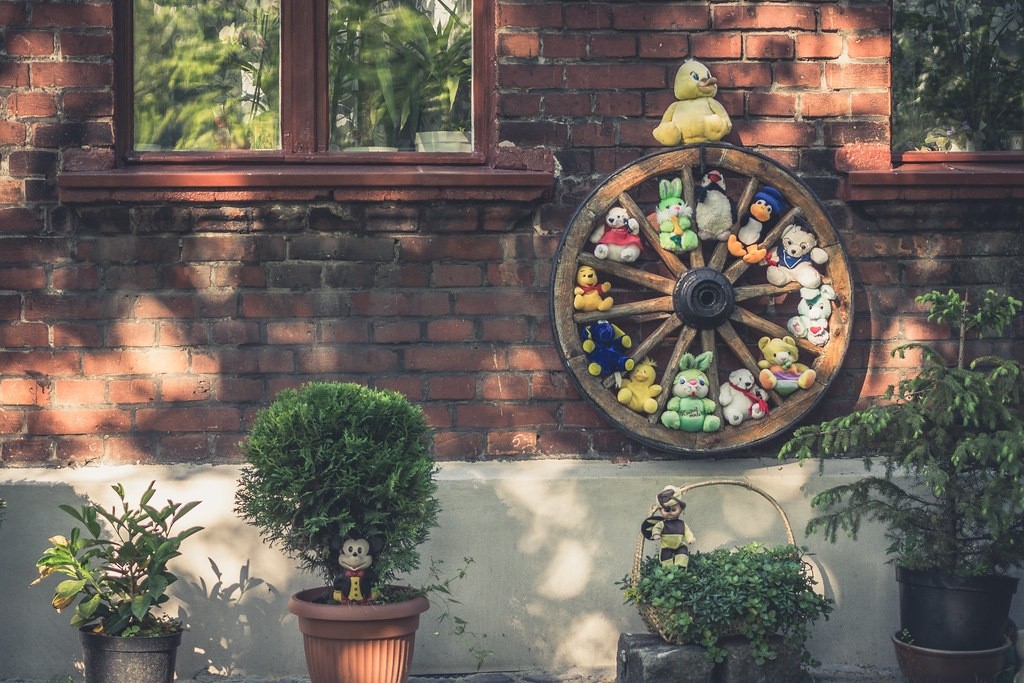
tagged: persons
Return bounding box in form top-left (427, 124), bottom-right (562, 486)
top-left (652, 485), bottom-right (696, 572)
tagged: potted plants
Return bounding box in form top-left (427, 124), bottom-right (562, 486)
top-left (29, 480), bottom-right (205, 682)
top-left (233, 380), bottom-right (492, 683)
top-left (778, 285), bottom-right (1024, 651)
top-left (613, 539), bottom-right (835, 671)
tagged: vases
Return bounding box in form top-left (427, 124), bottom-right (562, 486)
top-left (891, 631), bottom-right (1012, 683)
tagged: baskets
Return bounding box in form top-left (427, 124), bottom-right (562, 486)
top-left (632, 481), bottom-right (804, 644)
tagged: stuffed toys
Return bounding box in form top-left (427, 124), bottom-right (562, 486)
top-left (573, 167), bottom-right (836, 432)
top-left (653, 59), bottom-right (732, 146)
top-left (328, 532), bottom-right (385, 605)
